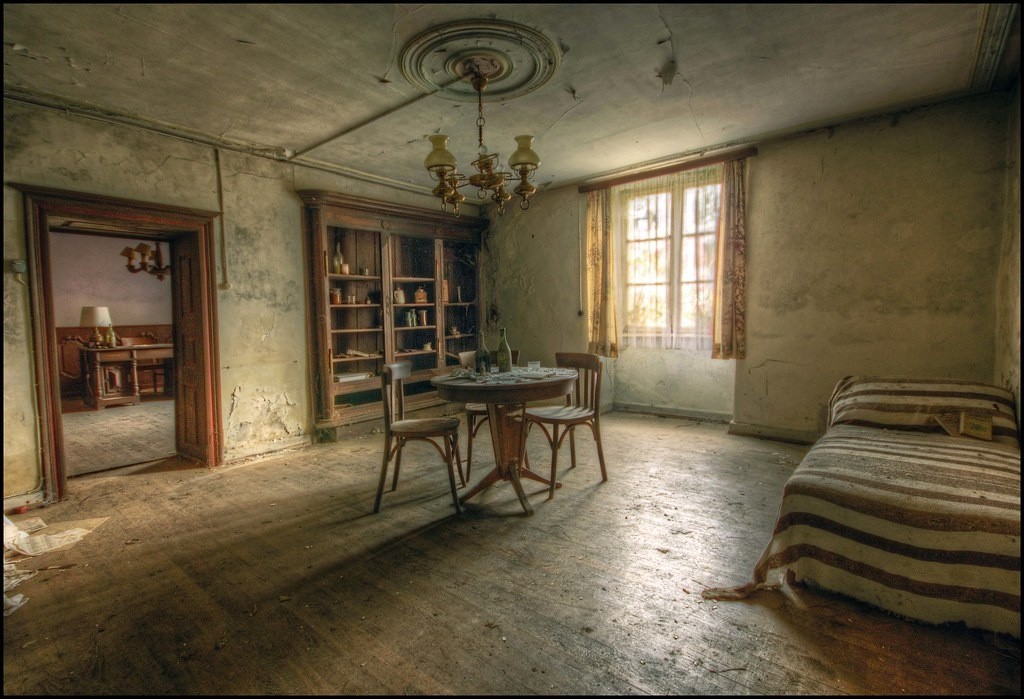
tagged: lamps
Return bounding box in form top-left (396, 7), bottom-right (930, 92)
top-left (120, 240), bottom-right (170, 281)
top-left (79, 305), bottom-right (112, 349)
top-left (424, 72), bottom-right (541, 218)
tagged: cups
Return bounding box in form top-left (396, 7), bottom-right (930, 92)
top-left (528, 361), bottom-right (540, 373)
top-left (347, 296), bottom-right (355, 304)
top-left (360, 269), bottom-right (369, 275)
top-left (340, 264), bottom-right (349, 275)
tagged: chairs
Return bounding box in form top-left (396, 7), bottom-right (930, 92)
top-left (120, 333), bottom-right (163, 393)
top-left (459, 349), bottom-right (531, 482)
top-left (523, 352), bottom-right (608, 500)
top-left (372, 358), bottom-right (467, 515)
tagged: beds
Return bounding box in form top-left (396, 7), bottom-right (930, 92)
top-left (753, 375), bottom-right (1021, 641)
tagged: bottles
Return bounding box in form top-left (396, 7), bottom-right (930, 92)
top-left (333, 243), bottom-right (344, 274)
top-left (106, 325), bottom-right (116, 347)
top-left (475, 330), bottom-right (491, 373)
top-left (498, 328), bottom-right (512, 372)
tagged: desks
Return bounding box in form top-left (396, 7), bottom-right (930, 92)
top-left (430, 367), bottom-right (578, 516)
top-left (79, 343), bottom-right (174, 410)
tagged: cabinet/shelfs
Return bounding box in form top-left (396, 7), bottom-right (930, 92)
top-left (295, 188), bottom-right (492, 443)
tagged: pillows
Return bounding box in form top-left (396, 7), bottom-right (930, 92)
top-left (832, 375), bottom-right (1019, 449)
